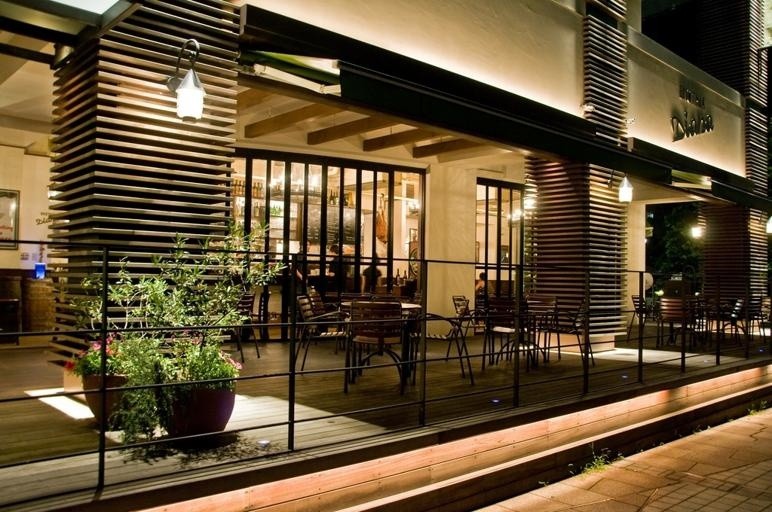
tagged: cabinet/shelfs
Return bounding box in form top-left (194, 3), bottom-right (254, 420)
top-left (231, 194), bottom-right (302, 241)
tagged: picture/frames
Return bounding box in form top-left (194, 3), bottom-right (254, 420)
top-left (0, 188), bottom-right (20, 250)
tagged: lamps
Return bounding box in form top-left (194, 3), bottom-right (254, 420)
top-left (759, 206), bottom-right (772, 234)
top-left (609, 161), bottom-right (634, 203)
top-left (168, 38), bottom-right (206, 120)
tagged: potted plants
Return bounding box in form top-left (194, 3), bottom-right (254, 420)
top-left (63, 256), bottom-right (168, 450)
top-left (155, 218), bottom-right (288, 438)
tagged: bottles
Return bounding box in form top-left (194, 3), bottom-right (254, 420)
top-left (396, 267), bottom-right (401, 287)
top-left (402, 269), bottom-right (408, 287)
top-left (230, 179), bottom-right (354, 218)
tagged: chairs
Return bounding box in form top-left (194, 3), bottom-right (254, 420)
top-left (626, 286), bottom-right (770, 350)
top-left (223, 284), bottom-right (596, 385)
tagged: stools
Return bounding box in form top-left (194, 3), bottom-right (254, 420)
top-left (0, 295), bottom-right (21, 345)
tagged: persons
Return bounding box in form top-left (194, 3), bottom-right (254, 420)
top-left (475, 271), bottom-right (495, 296)
top-left (328, 244), bottom-right (347, 291)
top-left (360, 255), bottom-right (383, 295)
top-left (292, 238), bottom-right (311, 292)
